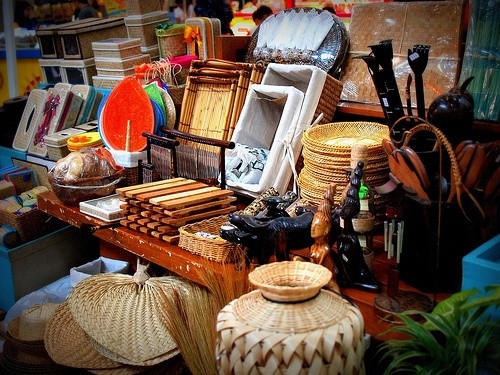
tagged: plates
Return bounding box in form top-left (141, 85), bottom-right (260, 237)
top-left (97, 75), bottom-right (176, 152)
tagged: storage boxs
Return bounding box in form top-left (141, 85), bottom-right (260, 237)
top-left (0, 224), bottom-right (98, 313)
top-left (394, 186), bottom-right (481, 294)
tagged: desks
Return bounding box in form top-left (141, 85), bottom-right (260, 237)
top-left (35, 192), bottom-right (461, 375)
top-left (335, 102), bottom-right (429, 123)
top-left (461, 234), bottom-right (500, 325)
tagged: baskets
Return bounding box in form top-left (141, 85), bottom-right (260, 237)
top-left (178, 213), bottom-right (248, 263)
top-left (47, 165), bottom-right (124, 206)
top-left (297, 121), bottom-right (391, 206)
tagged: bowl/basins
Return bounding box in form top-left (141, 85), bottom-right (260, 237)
top-left (47, 165), bottom-right (126, 206)
